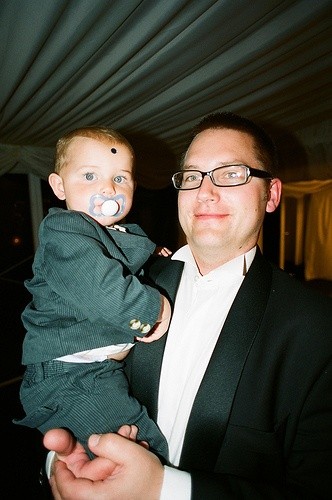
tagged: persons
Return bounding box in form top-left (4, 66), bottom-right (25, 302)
top-left (42, 113), bottom-right (332, 500)
top-left (11, 125), bottom-right (172, 466)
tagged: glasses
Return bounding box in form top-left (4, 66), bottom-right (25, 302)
top-left (170, 164), bottom-right (268, 190)
